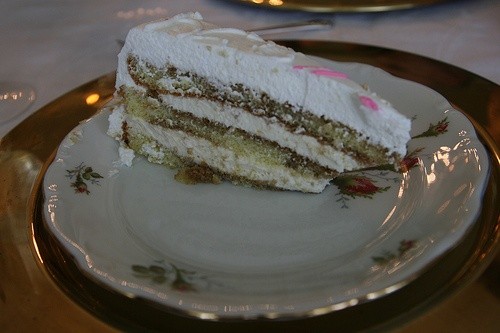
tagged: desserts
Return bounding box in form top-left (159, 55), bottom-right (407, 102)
top-left (105, 11), bottom-right (412, 193)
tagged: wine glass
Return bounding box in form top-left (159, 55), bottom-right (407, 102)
top-left (1, 80), bottom-right (35, 125)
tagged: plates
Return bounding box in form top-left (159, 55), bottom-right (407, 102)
top-left (40, 56), bottom-right (490, 318)
top-left (1, 41), bottom-right (500, 332)
